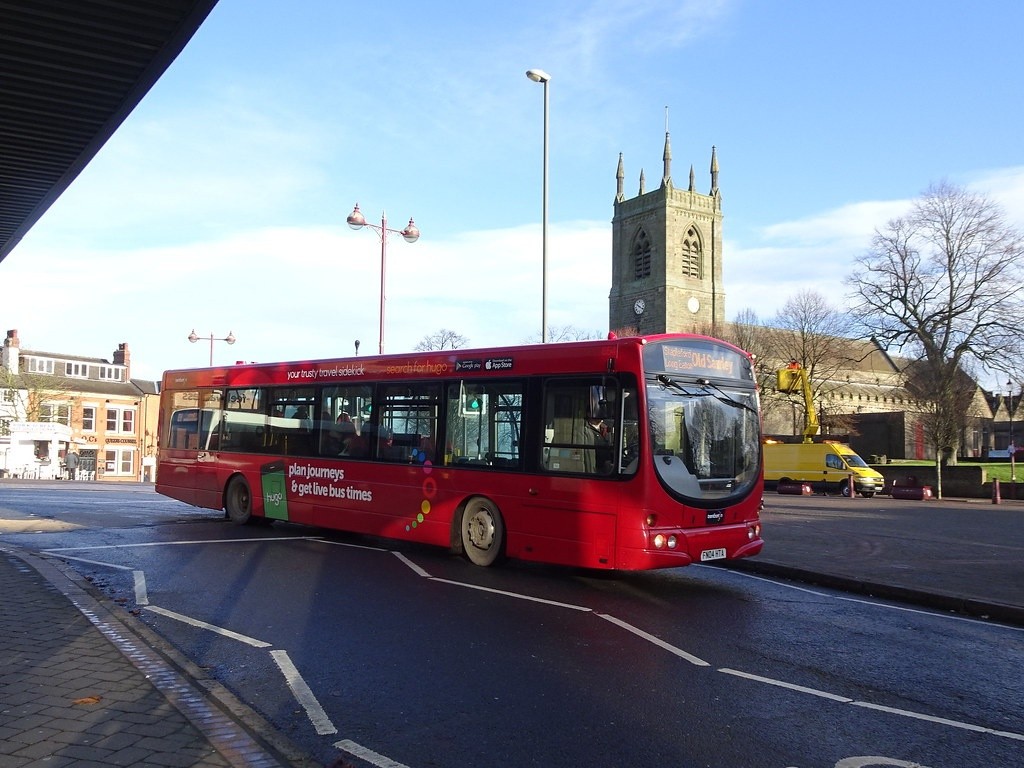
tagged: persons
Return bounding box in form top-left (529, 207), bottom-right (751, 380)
top-left (34, 446), bottom-right (40, 457)
top-left (575, 405), bottom-right (613, 475)
top-left (271, 406), bottom-right (390, 460)
top-left (63, 449), bottom-right (77, 480)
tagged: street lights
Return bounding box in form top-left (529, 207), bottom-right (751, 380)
top-left (346, 202), bottom-right (422, 353)
top-left (1006, 378), bottom-right (1017, 499)
top-left (186, 328), bottom-right (236, 367)
top-left (525, 67), bottom-right (551, 344)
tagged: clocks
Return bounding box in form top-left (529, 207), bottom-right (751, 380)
top-left (633, 299), bottom-right (646, 314)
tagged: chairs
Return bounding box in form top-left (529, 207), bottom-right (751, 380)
top-left (261, 407), bottom-right (613, 474)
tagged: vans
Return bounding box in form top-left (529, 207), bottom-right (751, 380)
top-left (760, 441), bottom-right (884, 496)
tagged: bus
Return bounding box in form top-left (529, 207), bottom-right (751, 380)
top-left (154, 331), bottom-right (765, 571)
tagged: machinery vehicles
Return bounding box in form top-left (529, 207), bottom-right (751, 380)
top-left (776, 361), bottom-right (824, 445)
